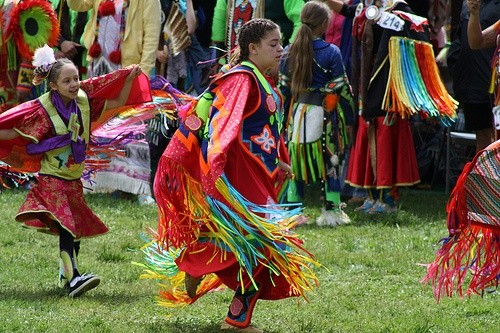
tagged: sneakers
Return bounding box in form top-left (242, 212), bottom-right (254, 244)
top-left (69, 272), bottom-right (100, 299)
top-left (59, 256), bottom-right (69, 287)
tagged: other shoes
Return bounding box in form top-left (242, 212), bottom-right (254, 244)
top-left (185, 272), bottom-right (202, 299)
top-left (221, 321), bottom-right (262, 333)
top-left (316, 211), bottom-right (351, 226)
top-left (353, 199), bottom-right (375, 210)
top-left (365, 200), bottom-right (397, 214)
top-left (291, 215), bottom-right (307, 224)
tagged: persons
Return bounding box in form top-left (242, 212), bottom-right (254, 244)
top-left (0, 58), bottom-right (143, 298)
top-left (0, 0), bottom-right (499, 226)
top-left (160, 18), bottom-right (295, 332)
